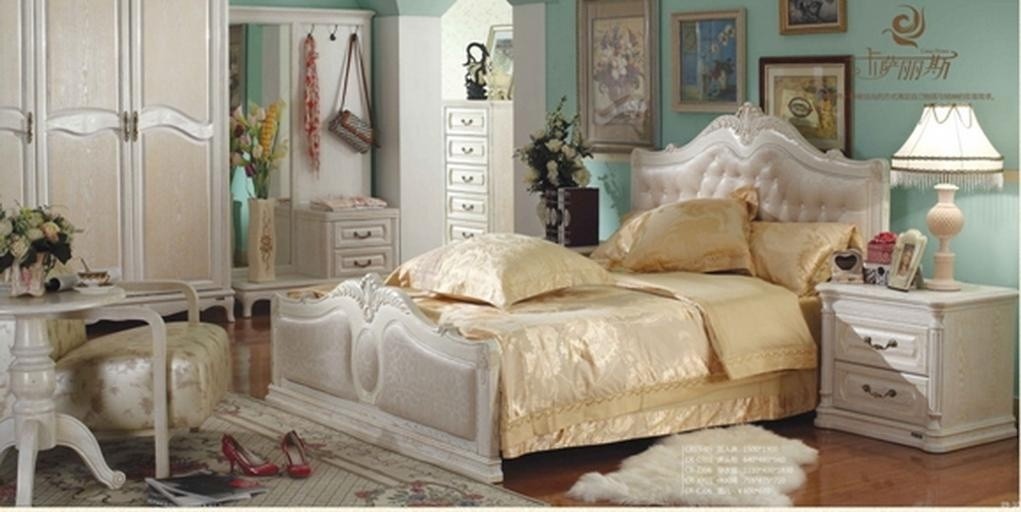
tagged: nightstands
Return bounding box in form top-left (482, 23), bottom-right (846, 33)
top-left (808, 274), bottom-right (1019, 456)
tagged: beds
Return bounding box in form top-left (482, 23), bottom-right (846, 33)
top-left (263, 99), bottom-right (894, 492)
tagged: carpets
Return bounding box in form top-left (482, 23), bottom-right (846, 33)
top-left (0, 392), bottom-right (559, 507)
top-left (563, 424), bottom-right (824, 506)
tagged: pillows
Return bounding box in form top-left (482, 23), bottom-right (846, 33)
top-left (590, 185), bottom-right (761, 276)
top-left (385, 229), bottom-right (620, 311)
top-left (751, 218), bottom-right (867, 298)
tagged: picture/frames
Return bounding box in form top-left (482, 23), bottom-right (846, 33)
top-left (887, 227), bottom-right (929, 292)
top-left (756, 53), bottom-right (854, 159)
top-left (671, 6), bottom-right (754, 116)
top-left (573, 0), bottom-right (662, 162)
top-left (486, 23), bottom-right (514, 102)
top-left (778, 0), bottom-right (848, 35)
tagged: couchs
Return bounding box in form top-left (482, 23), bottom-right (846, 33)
top-left (0, 238), bottom-right (236, 480)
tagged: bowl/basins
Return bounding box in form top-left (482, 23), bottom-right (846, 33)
top-left (79, 268), bottom-right (109, 289)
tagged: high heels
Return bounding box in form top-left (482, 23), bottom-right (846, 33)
top-left (280, 430), bottom-right (311, 478)
top-left (222, 433), bottom-right (279, 476)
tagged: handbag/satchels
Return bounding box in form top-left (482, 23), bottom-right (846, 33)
top-left (328, 110), bottom-right (374, 154)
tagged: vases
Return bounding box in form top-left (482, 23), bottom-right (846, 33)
top-left (247, 195), bottom-right (278, 283)
top-left (8, 253), bottom-right (46, 298)
top-left (542, 186), bottom-right (600, 246)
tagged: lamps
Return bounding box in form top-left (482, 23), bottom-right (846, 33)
top-left (887, 99), bottom-right (1006, 293)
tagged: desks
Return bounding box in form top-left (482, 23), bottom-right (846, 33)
top-left (0, 282), bottom-right (130, 507)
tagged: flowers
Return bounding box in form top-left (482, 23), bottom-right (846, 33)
top-left (510, 92), bottom-right (599, 198)
top-left (228, 95), bottom-right (291, 199)
top-left (0, 198), bottom-right (90, 274)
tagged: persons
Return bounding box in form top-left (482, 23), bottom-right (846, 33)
top-left (898, 249), bottom-right (912, 277)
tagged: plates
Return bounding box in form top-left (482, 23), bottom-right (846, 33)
top-left (72, 283), bottom-right (118, 296)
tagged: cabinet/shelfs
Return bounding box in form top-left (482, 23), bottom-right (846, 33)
top-left (0, 1), bottom-right (37, 216)
top-left (294, 206), bottom-right (400, 278)
top-left (37, 0), bottom-right (237, 326)
top-left (442, 101), bottom-right (515, 243)
